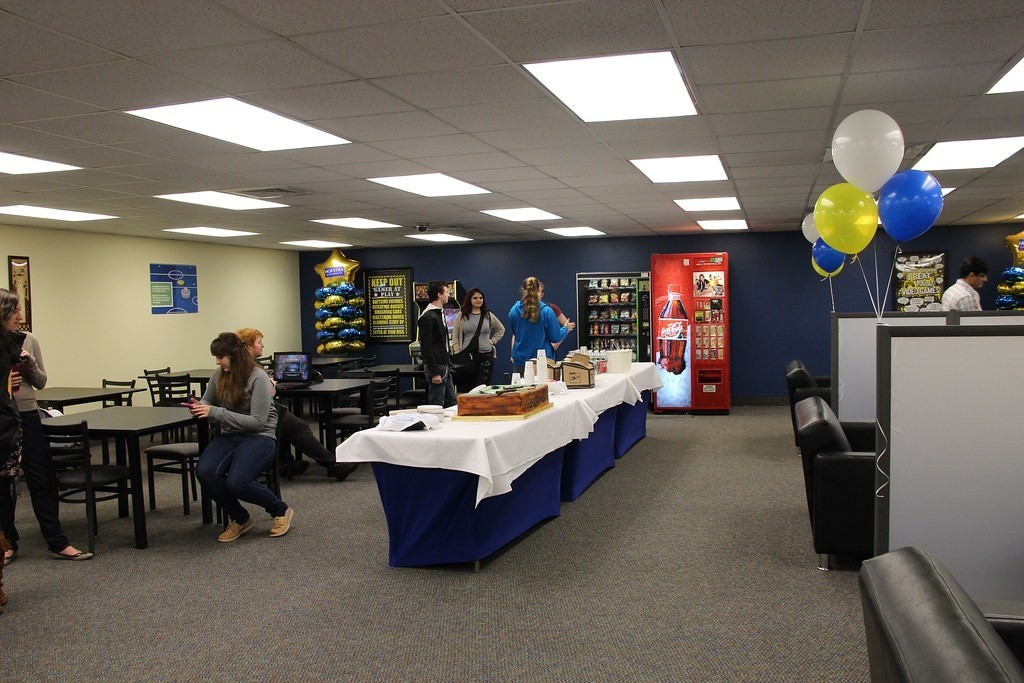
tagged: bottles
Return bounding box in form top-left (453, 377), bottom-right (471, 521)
top-left (658, 282), bottom-right (689, 375)
top-left (568, 349), bottom-right (607, 374)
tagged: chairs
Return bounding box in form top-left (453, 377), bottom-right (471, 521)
top-left (860, 546), bottom-right (1024, 683)
top-left (44, 356), bottom-right (400, 563)
top-left (784, 360), bottom-right (877, 570)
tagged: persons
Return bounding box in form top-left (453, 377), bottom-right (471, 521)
top-left (507, 277), bottom-right (575, 378)
top-left (188, 332), bottom-right (294, 542)
top-left (694, 274), bottom-right (724, 297)
top-left (417, 280), bottom-right (457, 409)
top-left (237, 327), bottom-right (361, 482)
top-left (452, 288), bottom-right (505, 394)
top-left (941, 256), bottom-right (989, 311)
top-left (0, 288), bottom-right (93, 614)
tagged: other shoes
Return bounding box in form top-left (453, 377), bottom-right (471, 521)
top-left (280, 460), bottom-right (309, 476)
top-left (50, 546), bottom-right (93, 560)
top-left (334, 462), bottom-right (358, 480)
top-left (3, 548), bottom-right (18, 566)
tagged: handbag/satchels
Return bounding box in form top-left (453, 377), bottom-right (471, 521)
top-left (450, 336), bottom-right (479, 362)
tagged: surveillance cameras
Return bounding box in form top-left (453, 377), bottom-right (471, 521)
top-left (417, 225), bottom-right (428, 232)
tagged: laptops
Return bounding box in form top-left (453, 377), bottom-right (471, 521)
top-left (273, 352), bottom-right (312, 390)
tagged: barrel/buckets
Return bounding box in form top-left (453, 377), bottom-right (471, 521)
top-left (605, 349), bottom-right (633, 374)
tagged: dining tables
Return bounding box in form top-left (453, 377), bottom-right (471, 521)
top-left (336, 361), bottom-right (664, 573)
top-left (21, 359), bottom-right (428, 551)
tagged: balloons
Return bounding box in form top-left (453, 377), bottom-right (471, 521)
top-left (831, 109), bottom-right (905, 193)
top-left (314, 250), bottom-right (365, 354)
top-left (811, 256), bottom-right (844, 277)
top-left (995, 230), bottom-right (1024, 311)
top-left (812, 237), bottom-right (846, 273)
top-left (813, 183), bottom-right (878, 254)
top-left (801, 212), bottom-right (820, 244)
top-left (877, 170), bottom-right (944, 242)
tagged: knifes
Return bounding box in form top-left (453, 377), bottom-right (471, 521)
top-left (496, 389), bottom-right (517, 395)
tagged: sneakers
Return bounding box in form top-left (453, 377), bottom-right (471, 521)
top-left (270, 507), bottom-right (294, 537)
top-left (219, 517), bottom-right (254, 543)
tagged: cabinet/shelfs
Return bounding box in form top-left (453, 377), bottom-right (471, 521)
top-left (576, 272), bottom-right (651, 366)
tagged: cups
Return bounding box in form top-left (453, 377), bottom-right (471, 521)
top-left (524, 360), bottom-right (534, 385)
top-left (536, 349), bottom-right (549, 386)
top-left (511, 373), bottom-right (521, 386)
